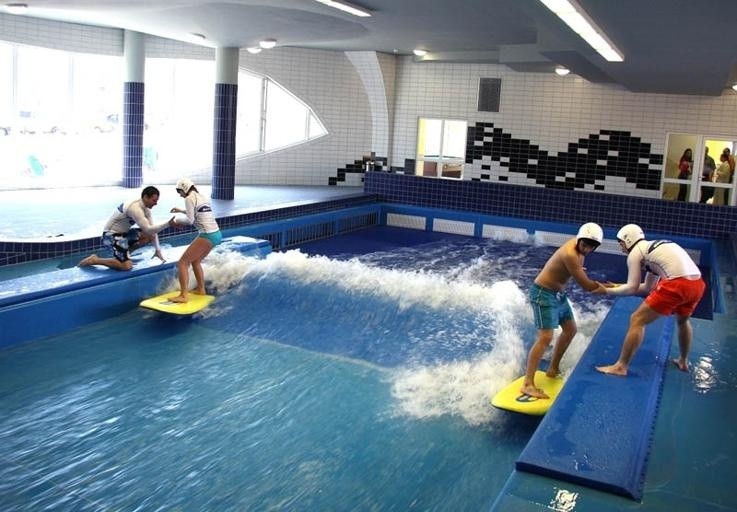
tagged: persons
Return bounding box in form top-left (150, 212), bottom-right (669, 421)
top-left (78, 186), bottom-right (178, 272)
top-left (167, 177), bottom-right (223, 303)
top-left (591, 224), bottom-right (707, 376)
top-left (520, 221), bottom-right (613, 399)
top-left (678, 146), bottom-right (735, 206)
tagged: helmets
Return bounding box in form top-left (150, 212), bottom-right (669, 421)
top-left (176, 178), bottom-right (194, 194)
top-left (616, 223), bottom-right (645, 252)
top-left (576, 223), bottom-right (603, 256)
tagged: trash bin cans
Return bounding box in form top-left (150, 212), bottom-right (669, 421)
top-left (366, 161), bottom-right (383, 171)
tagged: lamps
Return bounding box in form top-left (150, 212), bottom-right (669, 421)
top-left (246, 47), bottom-right (261, 54)
top-left (259, 38), bottom-right (277, 49)
top-left (317, 0), bottom-right (373, 18)
top-left (539, 0), bottom-right (624, 63)
top-left (555, 66), bottom-right (570, 76)
top-left (412, 49), bottom-right (428, 56)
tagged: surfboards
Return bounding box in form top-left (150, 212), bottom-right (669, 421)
top-left (490, 369), bottom-right (568, 417)
top-left (138, 287), bottom-right (214, 314)
